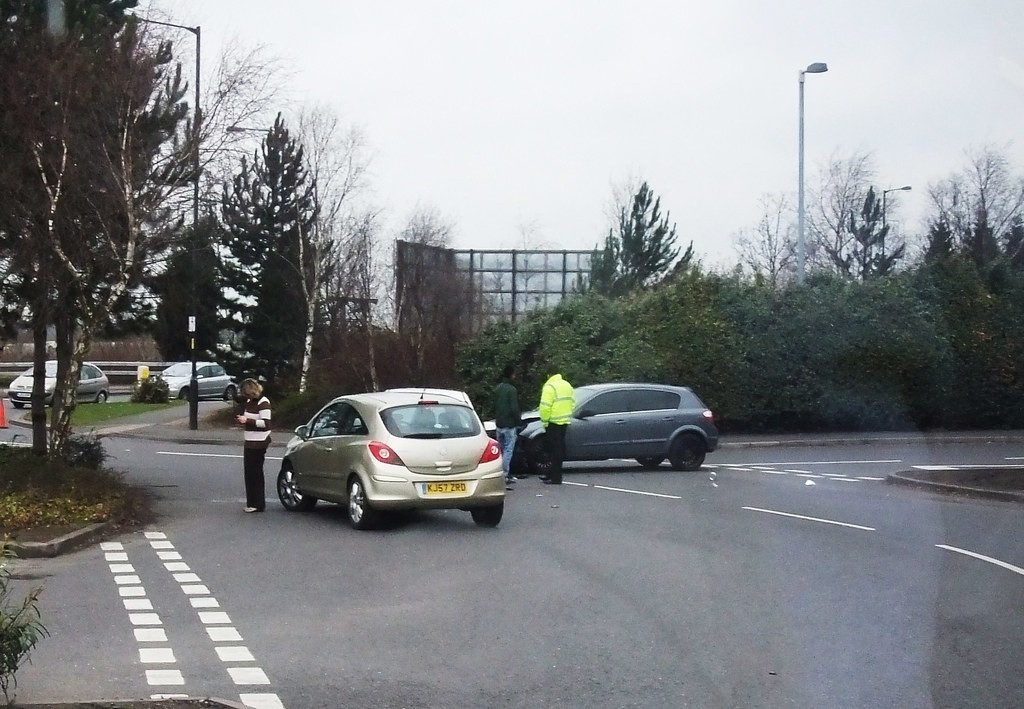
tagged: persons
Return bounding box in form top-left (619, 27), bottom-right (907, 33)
top-left (493, 366), bottom-right (523, 483)
top-left (539, 365), bottom-right (574, 484)
top-left (236, 378), bottom-right (272, 513)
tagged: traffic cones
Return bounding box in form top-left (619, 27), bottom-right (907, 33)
top-left (0, 396), bottom-right (10, 427)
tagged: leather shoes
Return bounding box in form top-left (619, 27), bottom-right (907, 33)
top-left (538, 476), bottom-right (561, 484)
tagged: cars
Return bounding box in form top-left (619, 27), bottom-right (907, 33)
top-left (148, 360), bottom-right (239, 401)
top-left (277, 392), bottom-right (506, 529)
top-left (484, 383), bottom-right (721, 478)
top-left (7, 360), bottom-right (109, 408)
top-left (383, 387), bottom-right (475, 410)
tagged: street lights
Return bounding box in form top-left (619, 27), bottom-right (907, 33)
top-left (125, 12), bottom-right (199, 430)
top-left (881, 185), bottom-right (912, 255)
top-left (797, 60), bottom-right (829, 283)
top-left (227, 126), bottom-right (285, 234)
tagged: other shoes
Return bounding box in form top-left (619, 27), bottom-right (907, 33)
top-left (242, 506), bottom-right (264, 513)
top-left (505, 479), bottom-right (516, 484)
